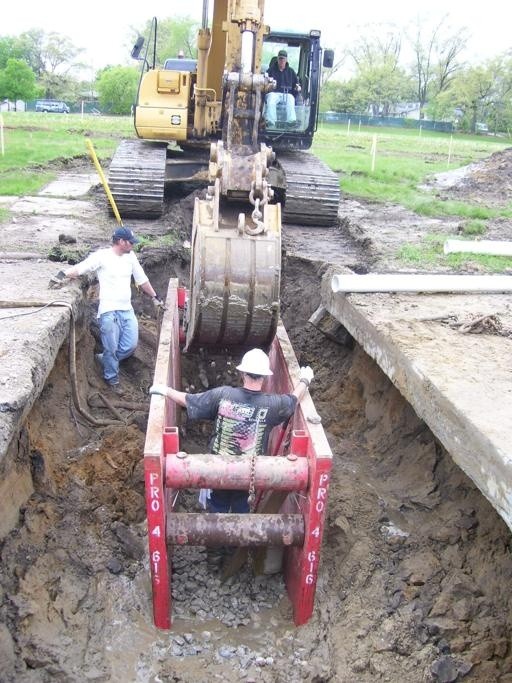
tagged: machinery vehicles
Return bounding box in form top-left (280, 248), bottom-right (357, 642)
top-left (107, 0), bottom-right (340, 351)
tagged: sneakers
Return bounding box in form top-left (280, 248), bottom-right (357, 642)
top-left (266, 119), bottom-right (296, 127)
top-left (95, 353), bottom-right (124, 395)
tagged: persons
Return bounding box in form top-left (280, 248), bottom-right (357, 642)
top-left (48, 227), bottom-right (168, 396)
top-left (262, 50), bottom-right (301, 129)
top-left (149, 348), bottom-right (314, 571)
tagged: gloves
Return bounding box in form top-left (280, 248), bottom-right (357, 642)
top-left (149, 384), bottom-right (168, 396)
top-left (50, 271), bottom-right (68, 289)
top-left (301, 366), bottom-right (314, 385)
top-left (152, 295), bottom-right (167, 311)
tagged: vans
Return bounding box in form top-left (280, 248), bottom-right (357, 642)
top-left (35, 101), bottom-right (69, 113)
top-left (475, 123), bottom-right (488, 133)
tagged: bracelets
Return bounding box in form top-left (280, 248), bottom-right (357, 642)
top-left (301, 377), bottom-right (309, 386)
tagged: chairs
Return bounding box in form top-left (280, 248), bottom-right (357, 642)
top-left (269, 57), bottom-right (277, 67)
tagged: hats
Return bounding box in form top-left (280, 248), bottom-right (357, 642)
top-left (113, 227), bottom-right (140, 244)
top-left (279, 50), bottom-right (287, 58)
top-left (235, 348), bottom-right (273, 375)
